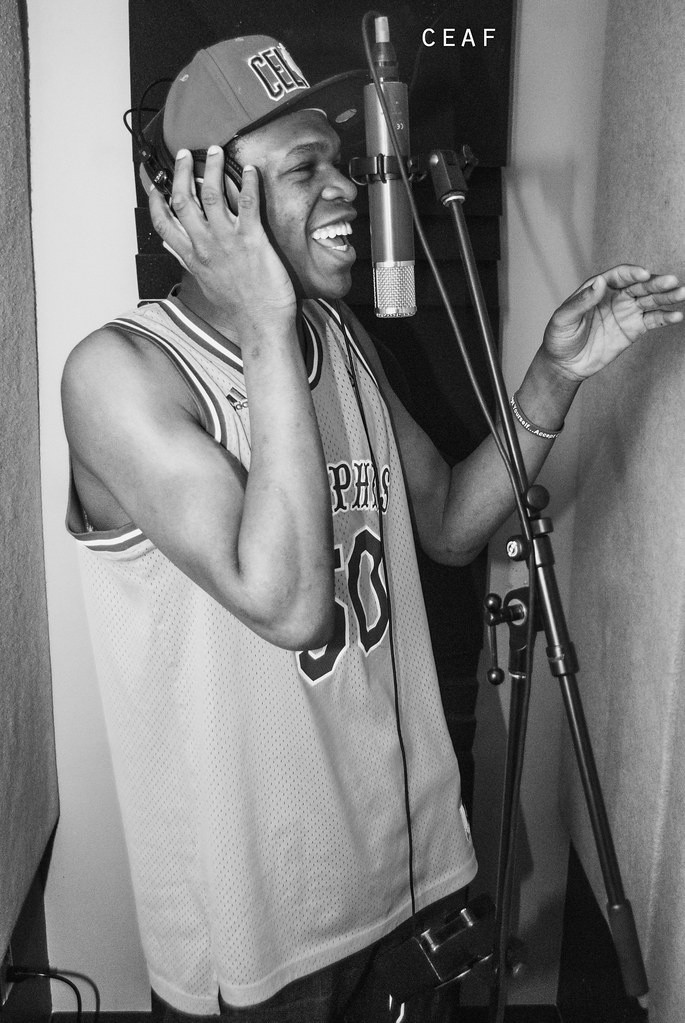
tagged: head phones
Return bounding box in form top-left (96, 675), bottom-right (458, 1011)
top-left (123, 77), bottom-right (245, 226)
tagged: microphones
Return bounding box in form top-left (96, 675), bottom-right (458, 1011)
top-left (364, 15), bottom-right (417, 318)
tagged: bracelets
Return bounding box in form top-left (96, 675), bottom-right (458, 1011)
top-left (509, 389), bottom-right (566, 438)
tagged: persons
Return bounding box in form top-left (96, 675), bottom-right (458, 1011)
top-left (62, 35), bottom-right (684, 1023)
